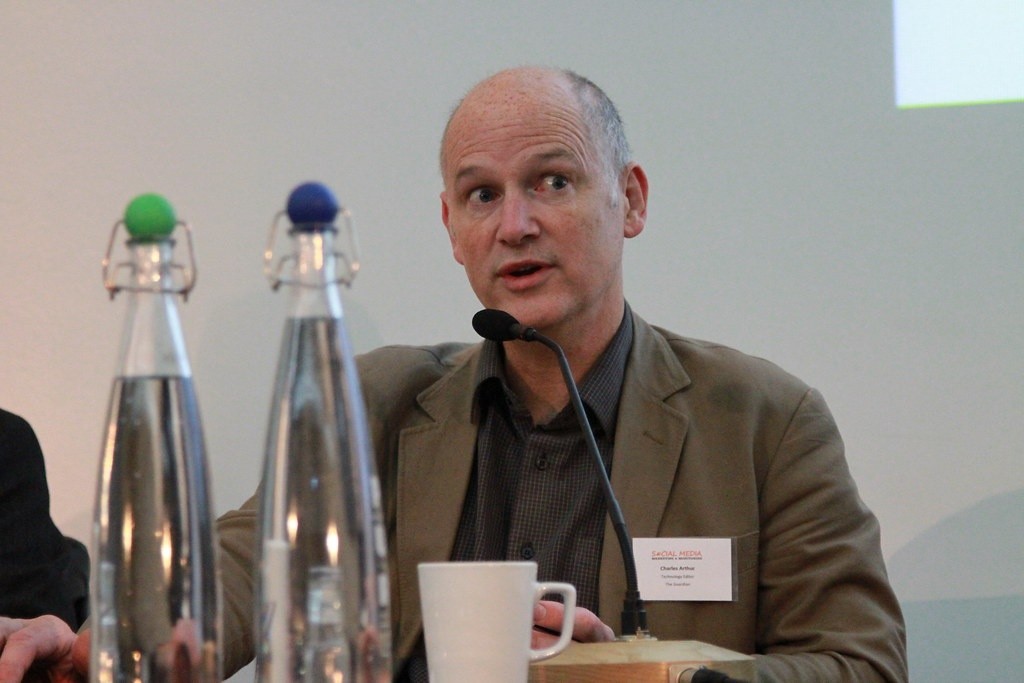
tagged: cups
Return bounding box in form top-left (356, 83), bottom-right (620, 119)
top-left (417, 560), bottom-right (577, 683)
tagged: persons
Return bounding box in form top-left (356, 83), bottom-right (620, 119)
top-left (0, 407), bottom-right (90, 683)
top-left (0, 63), bottom-right (907, 683)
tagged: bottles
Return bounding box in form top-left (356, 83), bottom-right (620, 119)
top-left (253, 181), bottom-right (391, 683)
top-left (88, 195), bottom-right (224, 683)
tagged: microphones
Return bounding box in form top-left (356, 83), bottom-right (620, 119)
top-left (471, 309), bottom-right (649, 639)
